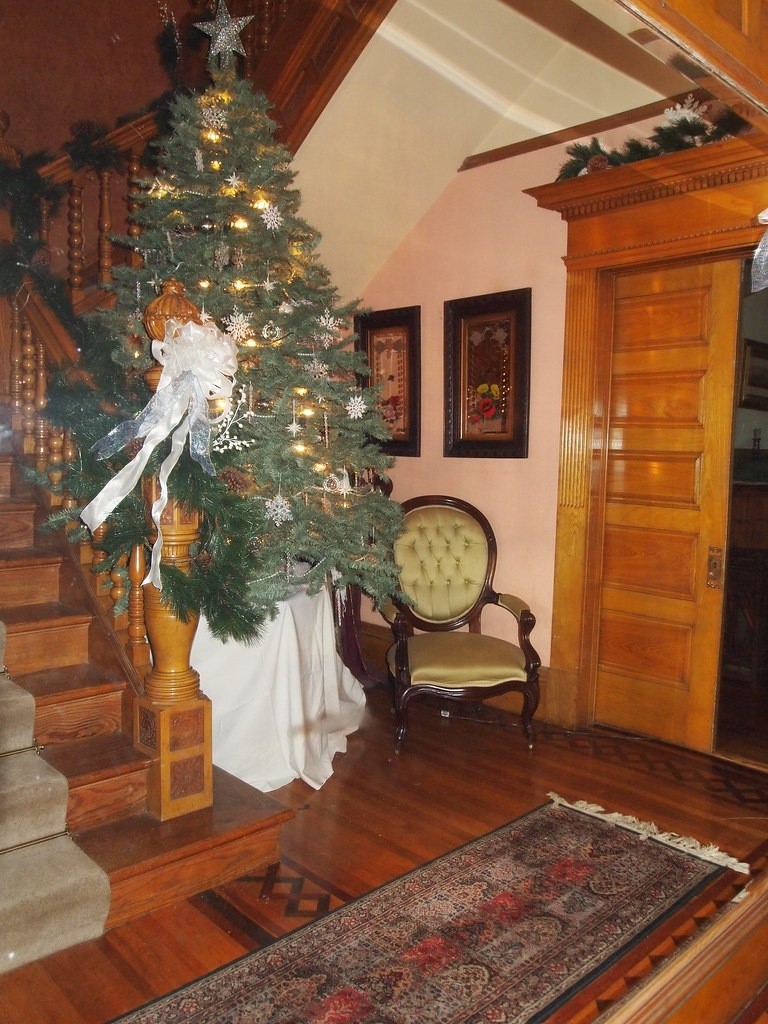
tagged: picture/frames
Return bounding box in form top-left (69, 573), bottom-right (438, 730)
top-left (443, 287), bottom-right (532, 458)
top-left (737, 337), bottom-right (768, 412)
top-left (353, 305), bottom-right (421, 457)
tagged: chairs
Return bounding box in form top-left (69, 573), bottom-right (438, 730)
top-left (376, 494), bottom-right (541, 754)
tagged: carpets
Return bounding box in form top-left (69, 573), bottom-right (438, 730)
top-left (0, 618), bottom-right (111, 974)
top-left (104, 790), bottom-right (768, 1024)
